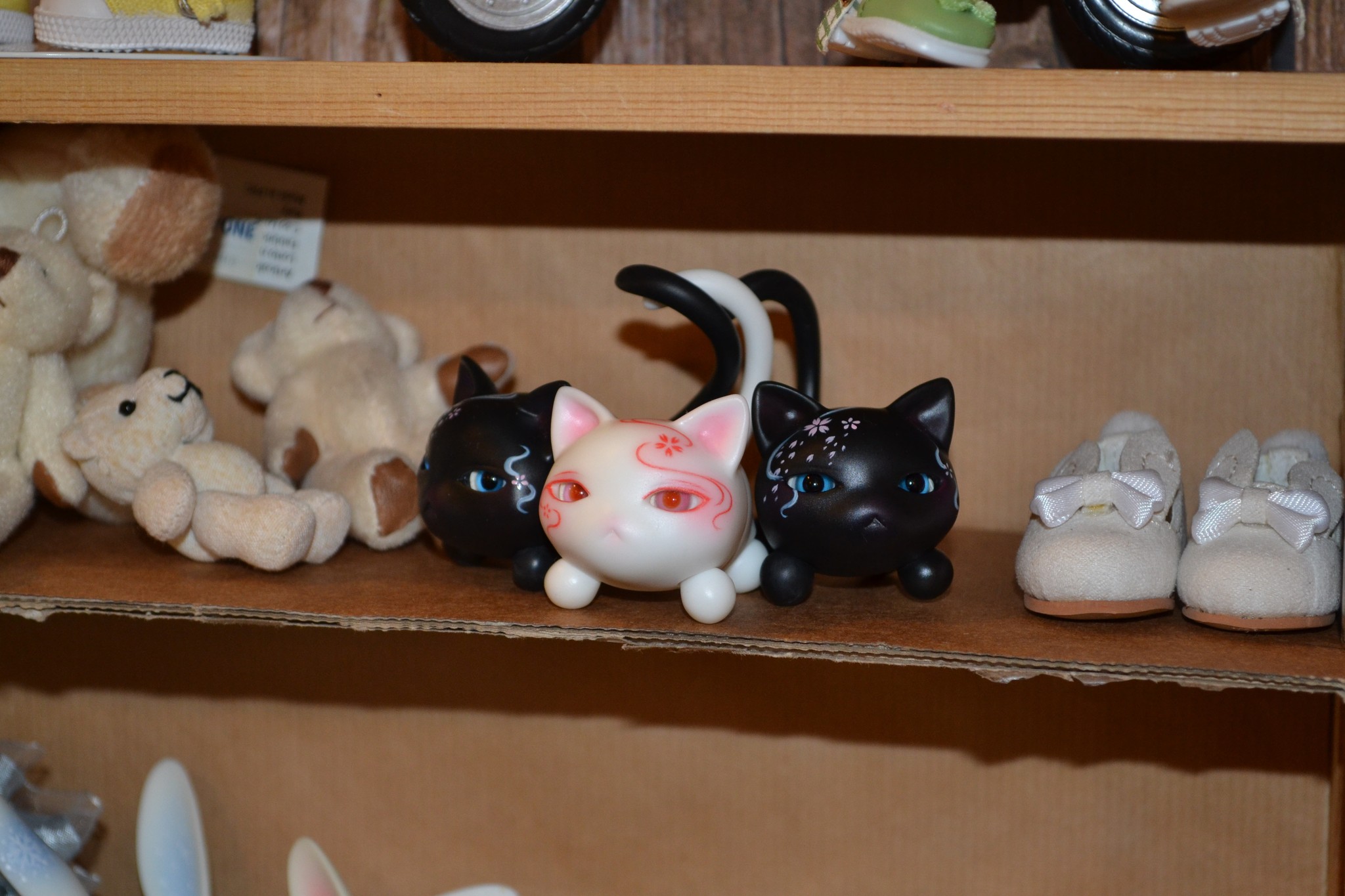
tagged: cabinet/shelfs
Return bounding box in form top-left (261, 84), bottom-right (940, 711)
top-left (0, 0), bottom-right (1345, 896)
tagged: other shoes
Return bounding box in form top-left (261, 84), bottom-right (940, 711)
top-left (1017, 409), bottom-right (1184, 619)
top-left (0, 0), bottom-right (41, 52)
top-left (832, 0), bottom-right (994, 68)
top-left (1179, 429), bottom-right (1345, 632)
top-left (31, 0), bottom-right (255, 55)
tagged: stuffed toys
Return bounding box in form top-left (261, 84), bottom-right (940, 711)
top-left (0, 124), bottom-right (226, 544)
top-left (60, 366), bottom-right (353, 572)
top-left (232, 276), bottom-right (515, 549)
